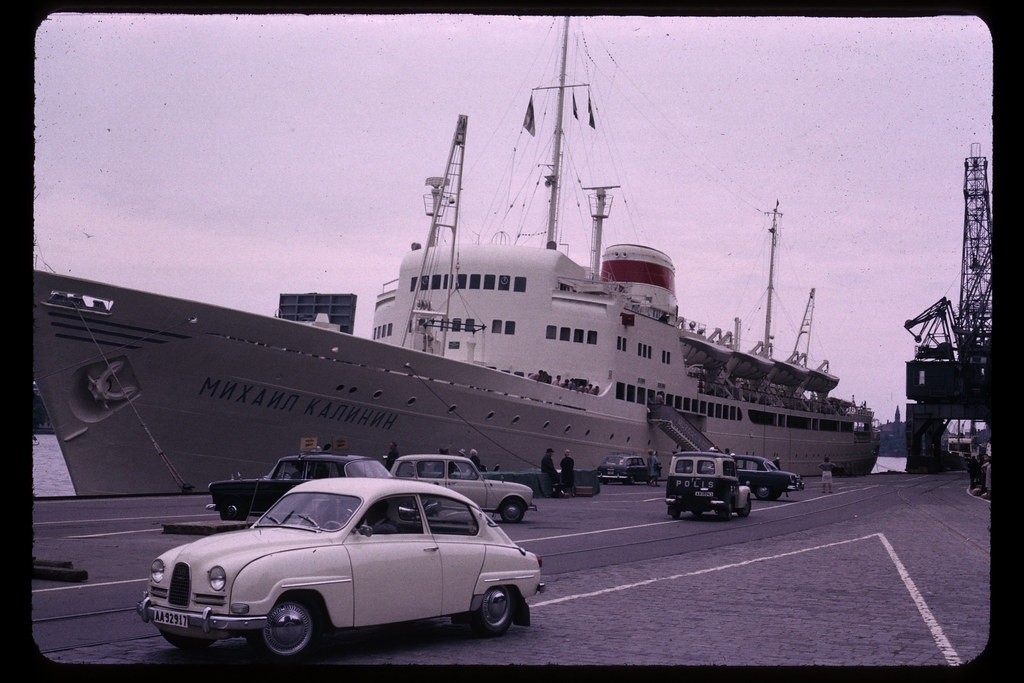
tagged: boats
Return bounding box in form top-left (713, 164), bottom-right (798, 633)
top-left (32, 16), bottom-right (883, 501)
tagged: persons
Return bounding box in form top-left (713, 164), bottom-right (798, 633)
top-left (818, 457), bottom-right (836, 493)
top-left (528, 370), bottom-right (599, 396)
top-left (656, 391), bottom-right (665, 404)
top-left (649, 449), bottom-right (676, 487)
top-left (967, 454), bottom-right (992, 491)
top-left (434, 446), bottom-right (480, 477)
top-left (724, 448), bottom-right (730, 455)
top-left (385, 441), bottom-right (399, 471)
top-left (542, 448), bottom-right (576, 498)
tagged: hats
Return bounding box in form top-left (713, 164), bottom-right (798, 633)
top-left (545, 448), bottom-right (554, 453)
top-left (457, 449), bottom-right (466, 456)
top-left (730, 453), bottom-right (735, 457)
top-left (470, 449), bottom-right (478, 456)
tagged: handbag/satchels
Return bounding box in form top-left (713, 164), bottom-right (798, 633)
top-left (653, 463), bottom-right (660, 471)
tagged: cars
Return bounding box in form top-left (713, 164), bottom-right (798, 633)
top-left (665, 451), bottom-right (752, 521)
top-left (390, 454), bottom-right (538, 525)
top-left (205, 449), bottom-right (443, 527)
top-left (135, 478), bottom-right (547, 667)
top-left (596, 452), bottom-right (656, 486)
top-left (705, 454), bottom-right (805, 502)
